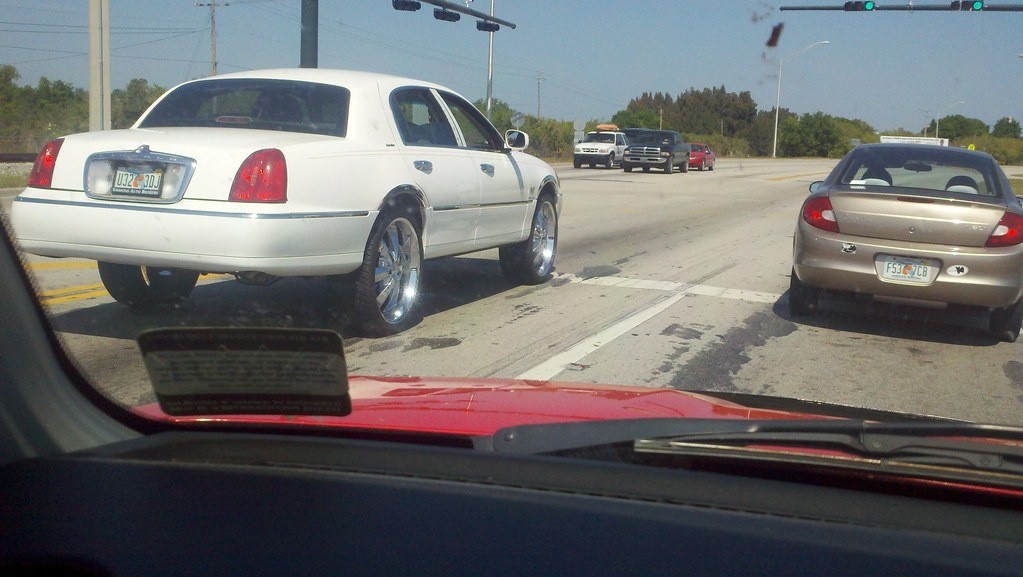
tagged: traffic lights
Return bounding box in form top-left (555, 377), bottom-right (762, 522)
top-left (951, 0), bottom-right (983, 11)
top-left (394, 0), bottom-right (421, 11)
top-left (434, 8), bottom-right (461, 22)
top-left (845, 0), bottom-right (874, 11)
top-left (477, 20), bottom-right (500, 32)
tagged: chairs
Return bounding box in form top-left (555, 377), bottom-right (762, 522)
top-left (252, 93), bottom-right (304, 122)
top-left (406, 121), bottom-right (435, 144)
top-left (862, 167), bottom-right (894, 187)
top-left (945, 176), bottom-right (979, 193)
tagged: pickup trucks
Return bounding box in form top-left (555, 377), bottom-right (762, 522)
top-left (622, 129), bottom-right (692, 174)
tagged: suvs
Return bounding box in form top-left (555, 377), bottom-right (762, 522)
top-left (573, 124), bottom-right (630, 170)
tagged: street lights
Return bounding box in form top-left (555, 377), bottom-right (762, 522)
top-left (772, 39), bottom-right (831, 158)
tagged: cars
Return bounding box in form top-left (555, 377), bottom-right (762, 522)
top-left (686, 142), bottom-right (716, 171)
top-left (788, 142), bottom-right (1023, 343)
top-left (620, 127), bottom-right (648, 139)
top-left (7, 68), bottom-right (563, 337)
